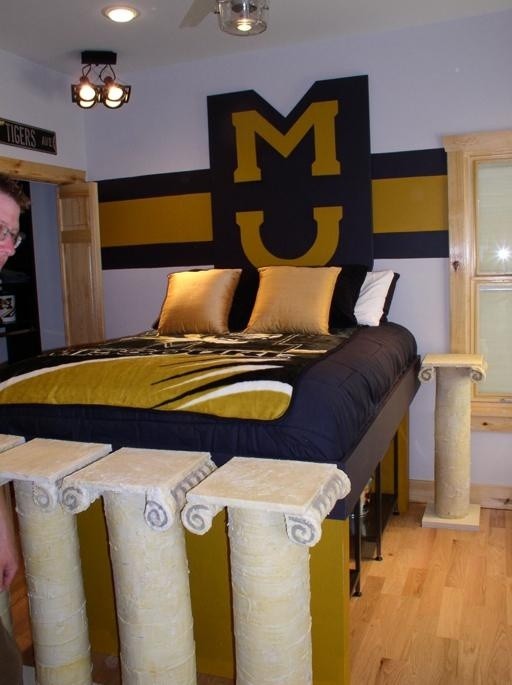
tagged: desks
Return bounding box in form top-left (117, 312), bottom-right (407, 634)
top-left (417, 352), bottom-right (488, 534)
top-left (0, 434), bottom-right (351, 685)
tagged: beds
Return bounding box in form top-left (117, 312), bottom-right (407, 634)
top-left (0, 322), bottom-right (422, 685)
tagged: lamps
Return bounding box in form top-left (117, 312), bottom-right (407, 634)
top-left (71, 50), bottom-right (132, 109)
top-left (214, 0), bottom-right (270, 36)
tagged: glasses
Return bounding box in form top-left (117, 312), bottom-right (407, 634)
top-left (0, 223), bottom-right (26, 249)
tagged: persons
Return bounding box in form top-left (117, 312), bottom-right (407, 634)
top-left (0, 173), bottom-right (33, 684)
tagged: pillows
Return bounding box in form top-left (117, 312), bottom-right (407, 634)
top-left (150, 265), bottom-right (400, 337)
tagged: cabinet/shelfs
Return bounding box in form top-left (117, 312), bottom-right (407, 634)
top-left (442, 125), bottom-right (512, 431)
